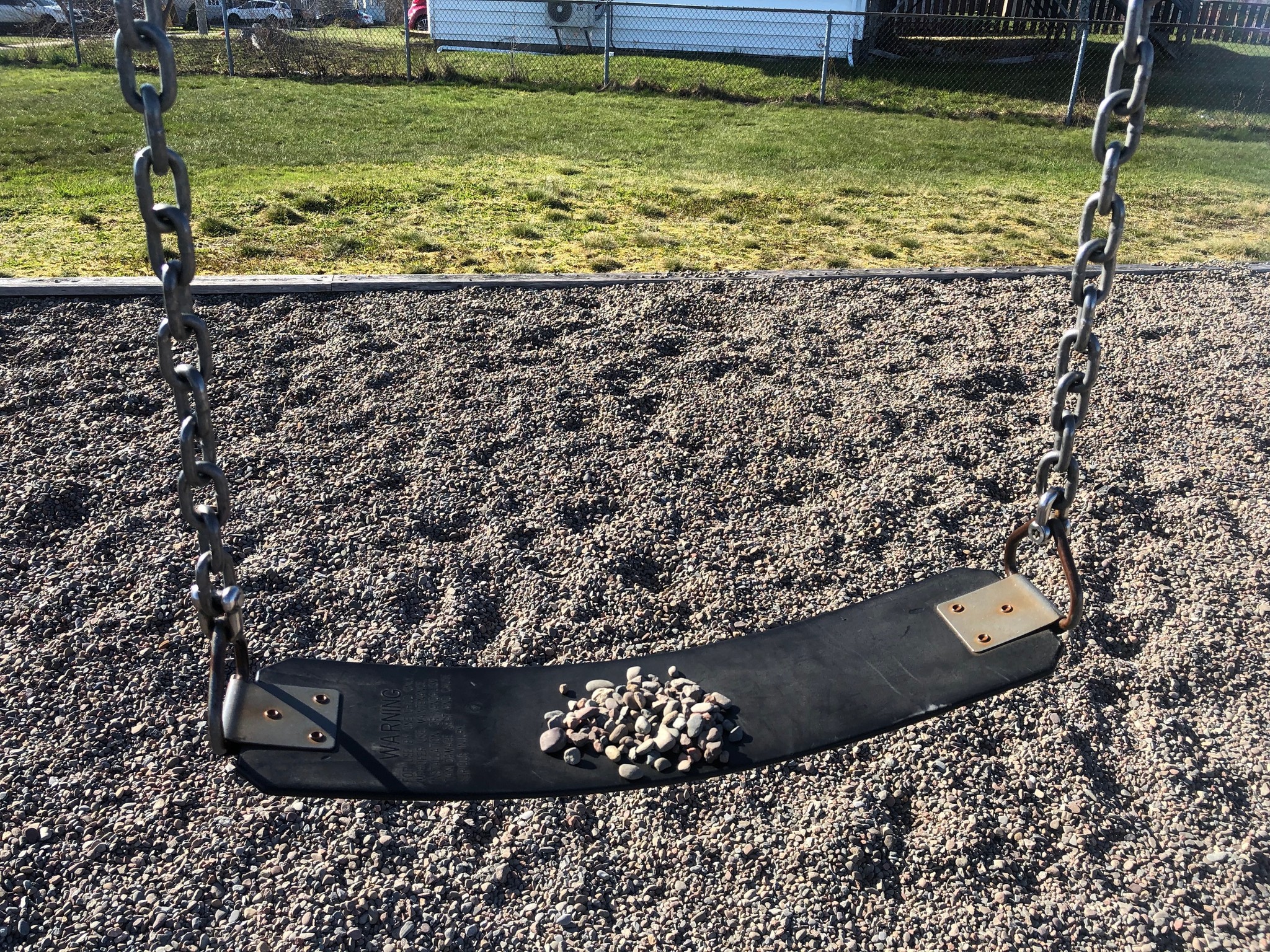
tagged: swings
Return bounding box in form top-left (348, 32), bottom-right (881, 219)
top-left (110, 0), bottom-right (1156, 803)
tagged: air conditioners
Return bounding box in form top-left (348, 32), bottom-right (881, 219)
top-left (544, 2), bottom-right (595, 28)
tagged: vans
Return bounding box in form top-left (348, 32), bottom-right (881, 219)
top-left (0, 0), bottom-right (69, 35)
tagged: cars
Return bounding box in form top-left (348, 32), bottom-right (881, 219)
top-left (408, 0), bottom-right (428, 32)
top-left (72, 9), bottom-right (108, 33)
top-left (227, 0), bottom-right (294, 28)
top-left (316, 8), bottom-right (374, 29)
